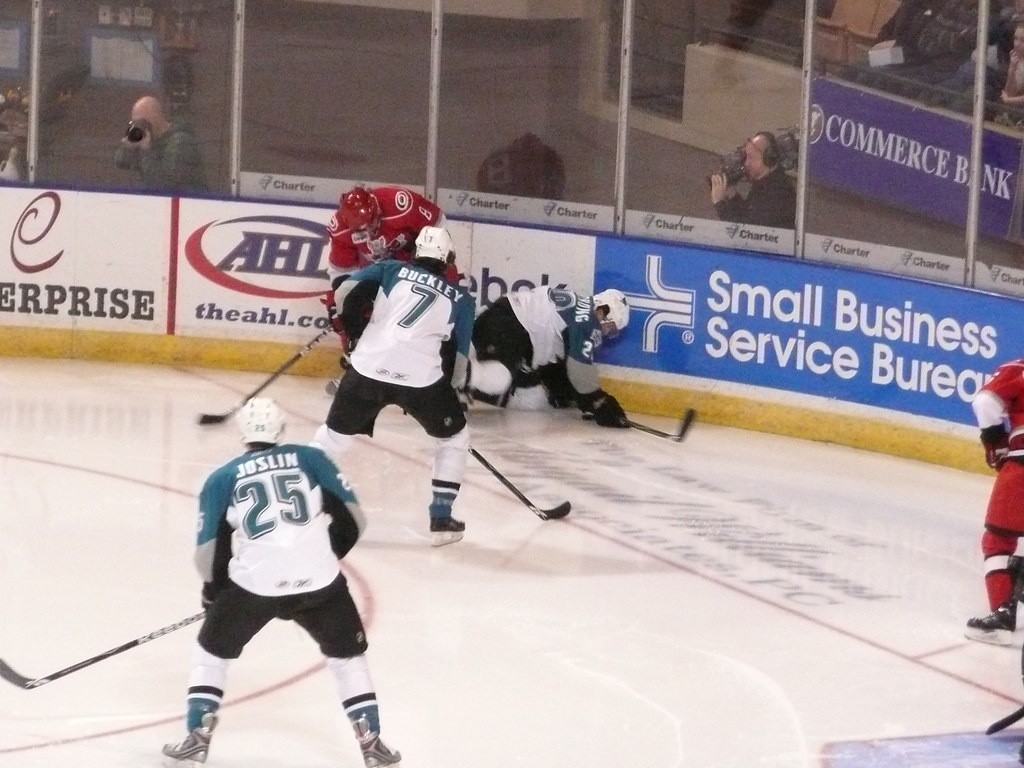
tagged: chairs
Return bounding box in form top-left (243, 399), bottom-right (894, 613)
top-left (839, 1), bottom-right (1015, 112)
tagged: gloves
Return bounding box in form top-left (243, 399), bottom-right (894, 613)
top-left (201, 580), bottom-right (222, 610)
top-left (594, 395), bottom-right (630, 429)
top-left (339, 338), bottom-right (356, 371)
top-left (548, 369), bottom-right (581, 408)
top-left (455, 388), bottom-right (473, 411)
top-left (979, 432), bottom-right (1010, 472)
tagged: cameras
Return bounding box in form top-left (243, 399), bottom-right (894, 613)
top-left (126, 119), bottom-right (153, 142)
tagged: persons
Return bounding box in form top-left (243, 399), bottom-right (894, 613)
top-left (456, 285), bottom-right (630, 428)
top-left (320, 185), bottom-right (460, 396)
top-left (836, 0), bottom-right (1024, 127)
top-left (711, 131), bottom-right (797, 230)
top-left (115, 96), bottom-right (209, 194)
top-left (479, 132), bottom-right (566, 200)
top-left (308, 225), bottom-right (471, 547)
top-left (964, 358), bottom-right (1024, 645)
top-left (160, 397), bottom-right (402, 768)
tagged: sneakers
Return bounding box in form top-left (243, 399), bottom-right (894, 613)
top-left (160, 712), bottom-right (220, 768)
top-left (352, 718), bottom-right (402, 768)
top-left (963, 596), bottom-right (1017, 645)
top-left (428, 504), bottom-right (466, 547)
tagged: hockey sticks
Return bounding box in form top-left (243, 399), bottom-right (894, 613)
top-left (0, 610), bottom-right (208, 690)
top-left (984, 705), bottom-right (1024, 737)
top-left (200, 325), bottom-right (336, 424)
top-left (620, 406), bottom-right (695, 444)
top-left (467, 443), bottom-right (573, 521)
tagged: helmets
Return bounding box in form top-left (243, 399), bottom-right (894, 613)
top-left (338, 186), bottom-right (381, 236)
top-left (238, 397), bottom-right (285, 446)
top-left (414, 226), bottom-right (457, 266)
top-left (593, 288), bottom-right (631, 330)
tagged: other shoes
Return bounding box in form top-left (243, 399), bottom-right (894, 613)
top-left (325, 376), bottom-right (342, 395)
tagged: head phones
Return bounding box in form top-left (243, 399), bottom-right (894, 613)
top-left (757, 131), bottom-right (781, 168)
